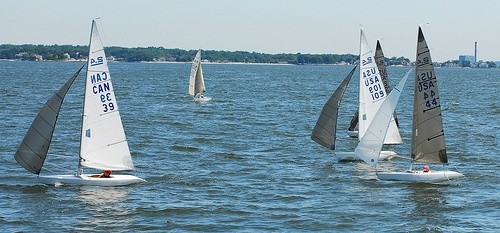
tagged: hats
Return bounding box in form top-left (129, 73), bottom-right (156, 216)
top-left (423, 166), bottom-right (430, 171)
top-left (104, 170), bottom-right (113, 175)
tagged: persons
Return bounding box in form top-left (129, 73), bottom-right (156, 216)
top-left (423, 166), bottom-right (431, 173)
top-left (98, 170), bottom-right (112, 178)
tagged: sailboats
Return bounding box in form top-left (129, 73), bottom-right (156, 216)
top-left (308, 31), bottom-right (406, 163)
top-left (11, 17), bottom-right (149, 188)
top-left (345, 38), bottom-right (401, 138)
top-left (187, 48), bottom-right (212, 104)
top-left (353, 24), bottom-right (466, 182)
